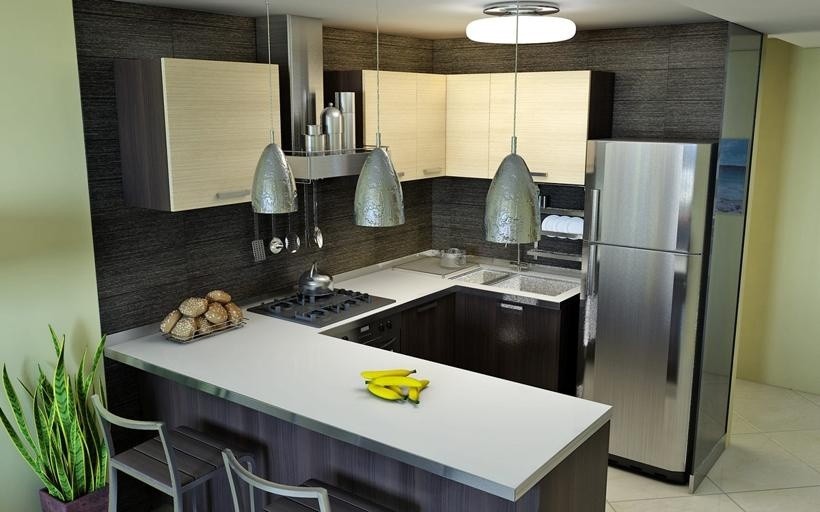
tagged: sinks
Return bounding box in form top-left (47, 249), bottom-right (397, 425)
top-left (448, 267), bottom-right (514, 286)
top-left (490, 275), bottom-right (580, 298)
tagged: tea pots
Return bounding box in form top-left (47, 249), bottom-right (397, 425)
top-left (298, 260), bottom-right (334, 296)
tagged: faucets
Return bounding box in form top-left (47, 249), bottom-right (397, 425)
top-left (505, 243), bottom-right (520, 265)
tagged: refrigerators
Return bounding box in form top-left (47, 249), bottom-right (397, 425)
top-left (577, 137), bottom-right (754, 496)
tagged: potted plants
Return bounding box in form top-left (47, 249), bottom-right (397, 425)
top-left (0, 322), bottom-right (115, 512)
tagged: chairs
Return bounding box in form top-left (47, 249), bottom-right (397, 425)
top-left (221, 447), bottom-right (392, 511)
top-left (93, 393), bottom-right (257, 512)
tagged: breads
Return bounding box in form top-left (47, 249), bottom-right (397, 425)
top-left (160, 289), bottom-right (245, 339)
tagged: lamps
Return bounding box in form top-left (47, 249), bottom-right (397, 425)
top-left (483, 4), bottom-right (542, 244)
top-left (352, 3), bottom-right (405, 228)
top-left (250, 1), bottom-right (298, 215)
top-left (466, 3), bottom-right (576, 45)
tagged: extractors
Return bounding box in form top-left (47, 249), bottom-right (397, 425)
top-left (257, 14), bottom-right (391, 181)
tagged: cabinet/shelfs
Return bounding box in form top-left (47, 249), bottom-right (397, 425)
top-left (116, 58), bottom-right (282, 211)
top-left (336, 69), bottom-right (406, 184)
top-left (455, 281), bottom-right (583, 398)
top-left (487, 70), bottom-right (614, 188)
top-left (445, 73), bottom-right (489, 181)
top-left (405, 70), bottom-right (448, 183)
top-left (399, 290), bottom-right (461, 368)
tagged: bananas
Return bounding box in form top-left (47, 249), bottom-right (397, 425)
top-left (359, 368), bottom-right (431, 403)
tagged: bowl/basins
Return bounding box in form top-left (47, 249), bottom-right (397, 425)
top-left (439, 247), bottom-right (466, 269)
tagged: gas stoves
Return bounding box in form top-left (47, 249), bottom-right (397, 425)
top-left (246, 287), bottom-right (396, 328)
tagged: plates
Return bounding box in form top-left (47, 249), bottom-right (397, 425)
top-left (540, 214), bottom-right (585, 241)
